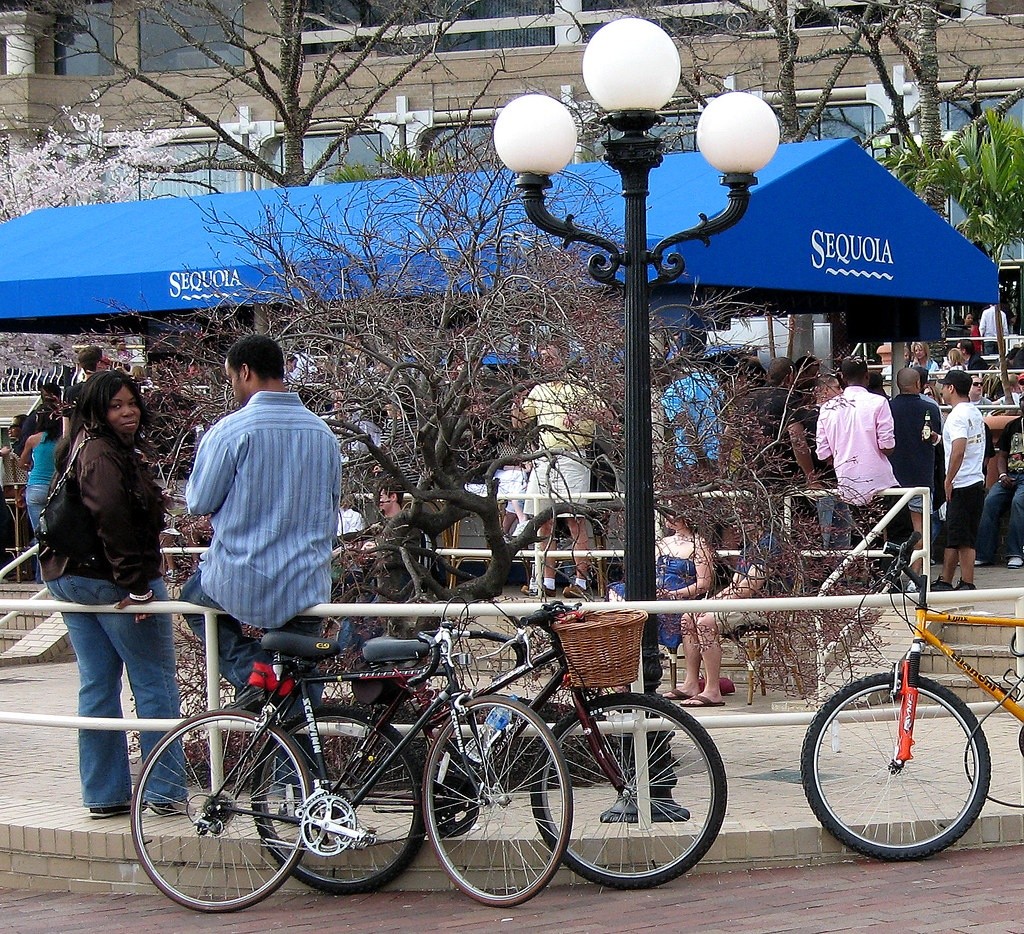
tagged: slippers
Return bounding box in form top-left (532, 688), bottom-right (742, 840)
top-left (662, 689), bottom-right (703, 700)
top-left (679, 694), bottom-right (726, 707)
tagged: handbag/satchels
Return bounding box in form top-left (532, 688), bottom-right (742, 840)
top-left (33, 434), bottom-right (105, 566)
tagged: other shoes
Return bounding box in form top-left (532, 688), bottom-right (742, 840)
top-left (8, 572), bottom-right (31, 582)
top-left (520, 583), bottom-right (557, 598)
top-left (905, 579), bottom-right (920, 593)
top-left (222, 683), bottom-right (269, 711)
top-left (562, 585), bottom-right (590, 598)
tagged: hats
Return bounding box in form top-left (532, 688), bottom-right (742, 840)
top-left (937, 370), bottom-right (974, 392)
top-left (1017, 374), bottom-right (1024, 381)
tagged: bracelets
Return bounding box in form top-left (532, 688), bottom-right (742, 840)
top-left (932, 434), bottom-right (941, 447)
top-left (999, 473), bottom-right (1007, 480)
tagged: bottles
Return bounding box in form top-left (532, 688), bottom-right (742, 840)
top-left (922, 410), bottom-right (933, 443)
top-left (943, 357), bottom-right (951, 371)
top-left (464, 696), bottom-right (518, 763)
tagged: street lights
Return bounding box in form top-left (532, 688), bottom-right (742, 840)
top-left (493, 20), bottom-right (778, 823)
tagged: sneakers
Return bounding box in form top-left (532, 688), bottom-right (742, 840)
top-left (89, 799), bottom-right (148, 819)
top-left (1006, 556), bottom-right (1024, 568)
top-left (930, 575), bottom-right (953, 592)
top-left (955, 578), bottom-right (976, 590)
top-left (974, 560), bottom-right (994, 566)
top-left (146, 798), bottom-right (198, 817)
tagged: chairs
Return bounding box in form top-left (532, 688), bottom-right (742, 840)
top-left (731, 541), bottom-right (808, 699)
top-left (657, 557), bottom-right (723, 694)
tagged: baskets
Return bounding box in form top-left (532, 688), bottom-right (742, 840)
top-left (551, 607), bottom-right (649, 687)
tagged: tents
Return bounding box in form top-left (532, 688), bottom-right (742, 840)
top-left (0, 142), bottom-right (1000, 321)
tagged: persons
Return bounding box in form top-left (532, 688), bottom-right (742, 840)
top-left (374, 402), bottom-right (429, 492)
top-left (494, 436), bottom-right (530, 562)
top-left (817, 358), bottom-right (915, 592)
top-left (866, 341), bottom-right (1024, 415)
top-left (654, 331), bottom-right (854, 586)
top-left (286, 340), bottom-right (390, 463)
top-left (336, 492), bottom-right (369, 543)
top-left (511, 337), bottom-right (616, 595)
top-left (605, 495), bottom-right (715, 651)
top-left (980, 304), bottom-right (1009, 354)
top-left (661, 500), bottom-right (797, 710)
top-left (509, 345), bottom-right (617, 598)
top-left (351, 478), bottom-right (435, 602)
top-left (958, 395), bottom-right (1024, 568)
top-left (886, 369), bottom-right (941, 577)
top-left (131, 366), bottom-right (149, 394)
top-left (0, 346), bottom-right (108, 581)
top-left (920, 372), bottom-right (984, 591)
top-left (42, 369), bottom-right (198, 819)
top-left (179, 335), bottom-right (344, 824)
top-left (960, 314), bottom-right (982, 355)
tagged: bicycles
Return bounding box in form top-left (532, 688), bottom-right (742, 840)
top-left (798, 530), bottom-right (1024, 862)
top-left (250, 599), bottom-right (729, 895)
top-left (131, 596), bottom-right (574, 910)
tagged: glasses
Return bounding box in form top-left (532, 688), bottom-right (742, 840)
top-left (973, 381), bottom-right (983, 387)
top-left (9, 423), bottom-right (21, 430)
top-left (1019, 380), bottom-right (1024, 385)
